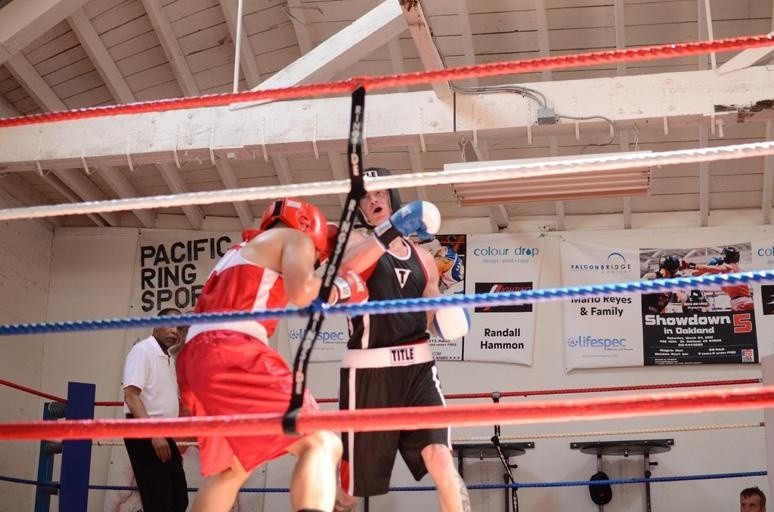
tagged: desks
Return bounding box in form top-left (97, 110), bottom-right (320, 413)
top-left (450, 440), bottom-right (671, 512)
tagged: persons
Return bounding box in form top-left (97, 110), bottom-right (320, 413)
top-left (174, 195), bottom-right (371, 511)
top-left (738, 486), bottom-right (767, 512)
top-left (640, 254), bottom-right (722, 315)
top-left (121, 308), bottom-right (189, 511)
top-left (332, 166), bottom-right (471, 511)
top-left (678, 247), bottom-right (754, 313)
top-left (419, 239), bottom-right (463, 295)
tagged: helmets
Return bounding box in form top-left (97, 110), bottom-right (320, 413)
top-left (660, 255), bottom-right (679, 272)
top-left (353, 165), bottom-right (402, 227)
top-left (260, 197), bottom-right (332, 269)
top-left (720, 246), bottom-right (740, 264)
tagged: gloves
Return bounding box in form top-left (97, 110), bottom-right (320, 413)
top-left (679, 259), bottom-right (688, 271)
top-left (433, 305), bottom-right (471, 341)
top-left (329, 269), bottom-right (368, 307)
top-left (708, 256), bottom-right (724, 266)
top-left (317, 223), bottom-right (339, 269)
top-left (372, 200), bottom-right (442, 250)
